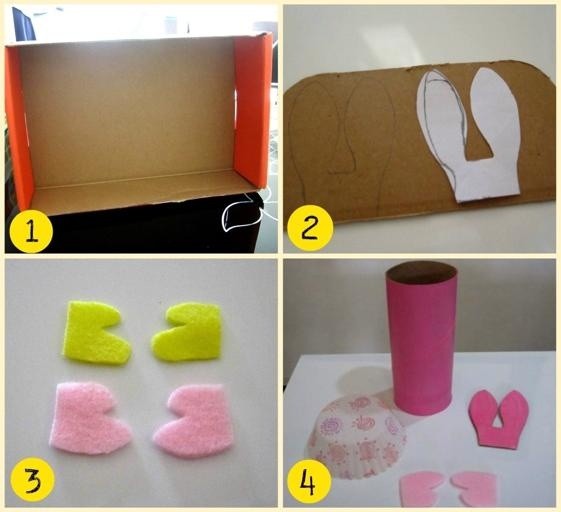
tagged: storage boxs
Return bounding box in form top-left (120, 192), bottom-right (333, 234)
top-left (3, 32), bottom-right (273, 217)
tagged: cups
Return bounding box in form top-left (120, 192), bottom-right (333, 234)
top-left (385, 261), bottom-right (457, 416)
